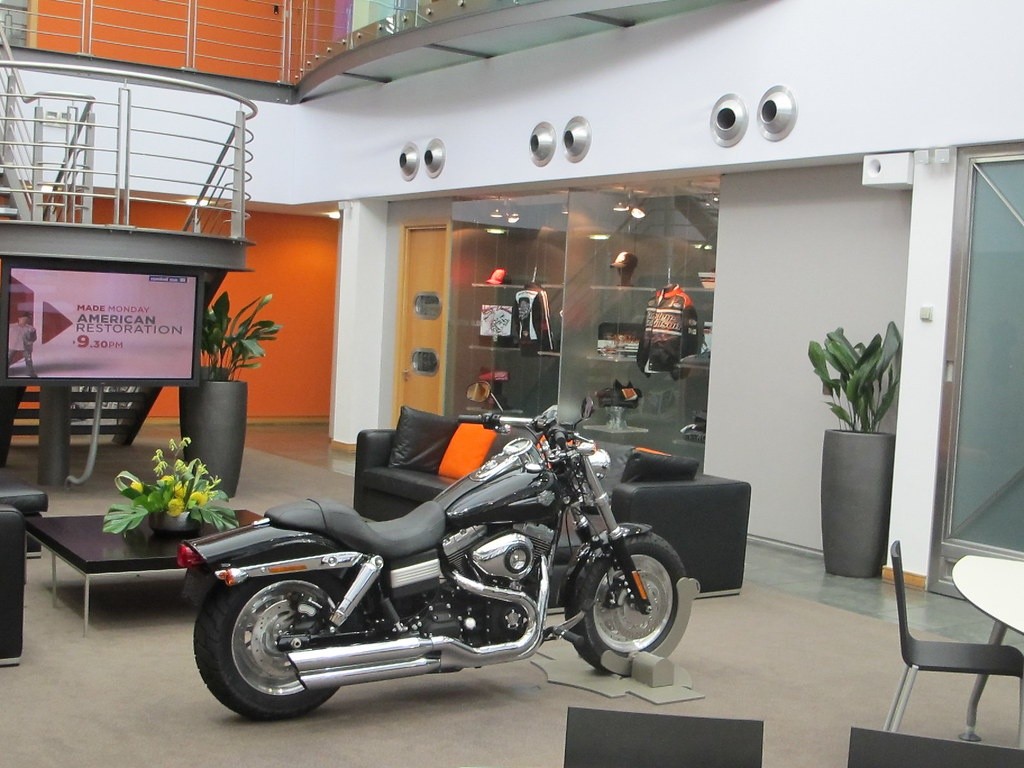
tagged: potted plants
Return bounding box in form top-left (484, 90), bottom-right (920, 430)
top-left (181, 291), bottom-right (285, 498)
top-left (807, 321), bottom-right (903, 576)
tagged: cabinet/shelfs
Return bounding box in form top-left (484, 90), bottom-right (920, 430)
top-left (582, 283), bottom-right (714, 376)
top-left (468, 280), bottom-right (564, 360)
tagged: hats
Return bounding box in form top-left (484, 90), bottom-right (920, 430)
top-left (485, 268), bottom-right (512, 284)
top-left (610, 251), bottom-right (638, 269)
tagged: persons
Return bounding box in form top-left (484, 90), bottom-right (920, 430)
top-left (9, 312), bottom-right (36, 376)
top-left (637, 284), bottom-right (700, 383)
top-left (509, 282), bottom-right (555, 356)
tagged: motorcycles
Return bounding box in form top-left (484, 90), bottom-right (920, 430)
top-left (177, 378), bottom-right (694, 725)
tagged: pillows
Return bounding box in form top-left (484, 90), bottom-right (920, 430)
top-left (536, 427), bottom-right (668, 510)
top-left (622, 453), bottom-right (699, 483)
top-left (439, 422), bottom-right (497, 479)
top-left (481, 430), bottom-right (545, 466)
top-left (387, 405), bottom-right (461, 472)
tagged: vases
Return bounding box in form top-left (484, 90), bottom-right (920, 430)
top-left (149, 511), bottom-right (201, 532)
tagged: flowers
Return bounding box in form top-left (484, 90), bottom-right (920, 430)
top-left (102, 434), bottom-right (240, 532)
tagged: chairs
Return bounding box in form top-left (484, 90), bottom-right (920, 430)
top-left (891, 540), bottom-right (1024, 749)
top-left (563, 707), bottom-right (764, 768)
top-left (845, 726), bottom-right (1024, 767)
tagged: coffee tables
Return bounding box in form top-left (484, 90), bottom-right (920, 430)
top-left (25, 511), bottom-right (266, 638)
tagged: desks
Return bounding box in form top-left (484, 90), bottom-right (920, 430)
top-left (953, 555), bottom-right (1024, 752)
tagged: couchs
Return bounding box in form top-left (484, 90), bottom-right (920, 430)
top-left (0, 463), bottom-right (50, 666)
top-left (354, 428), bottom-right (752, 600)
